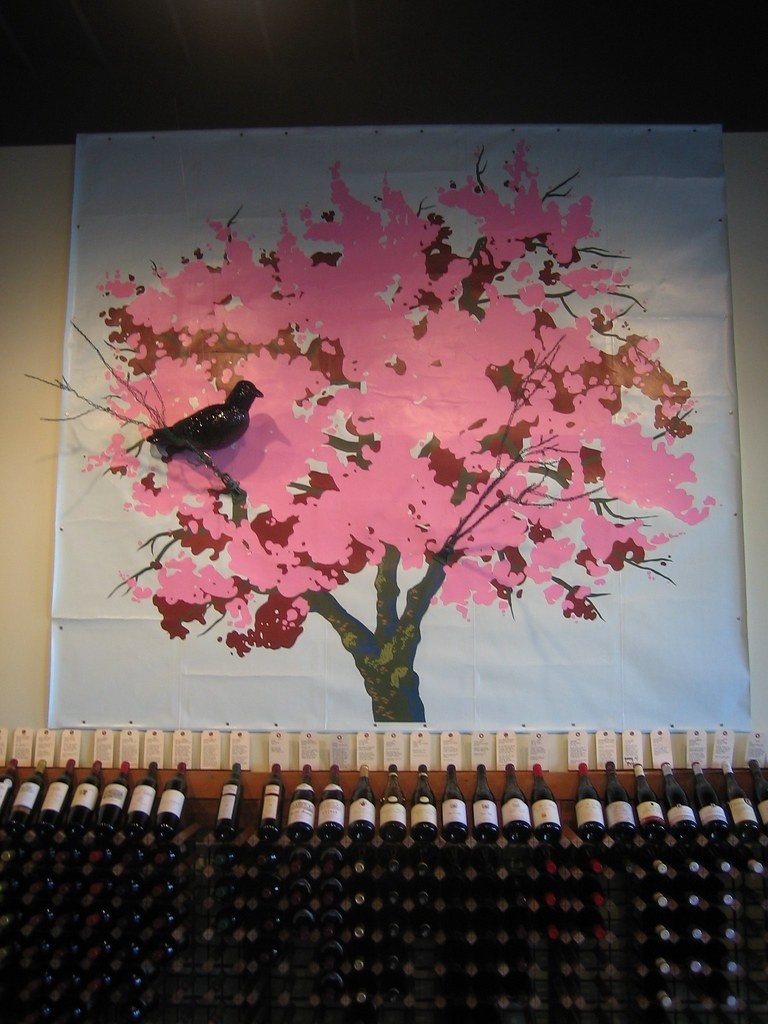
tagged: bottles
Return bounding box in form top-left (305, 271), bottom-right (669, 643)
top-left (0, 756), bottom-right (768, 1024)
